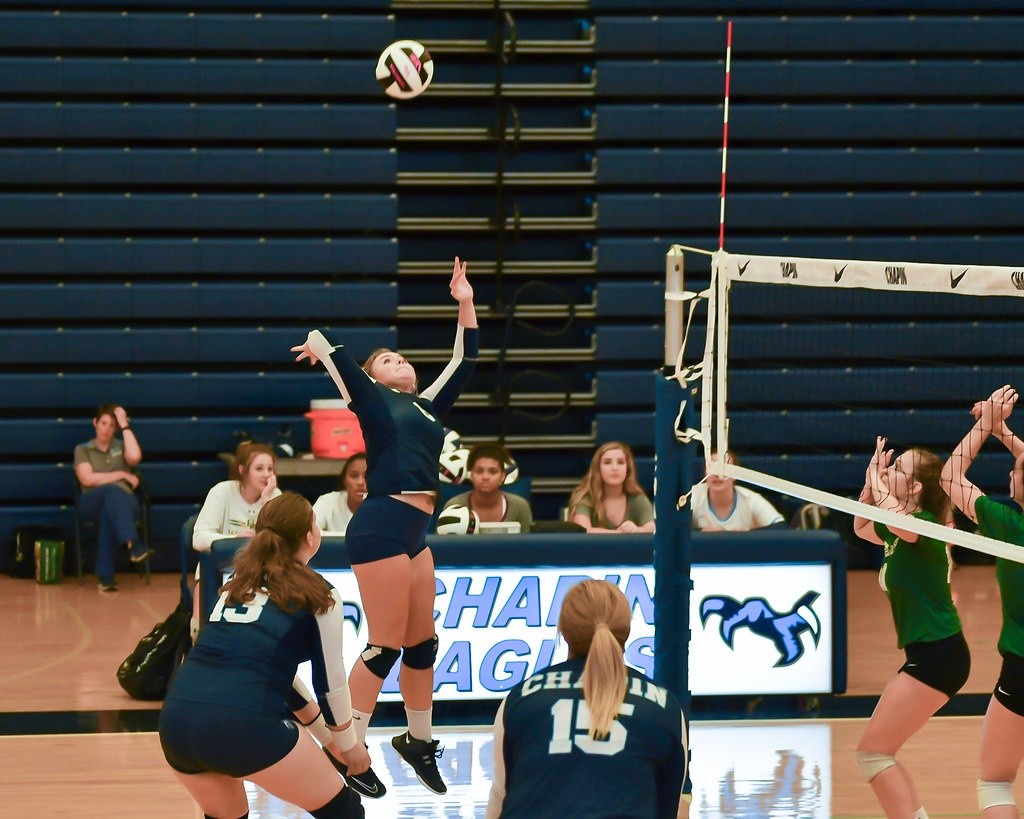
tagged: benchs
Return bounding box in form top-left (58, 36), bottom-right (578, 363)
top-left (0, 0), bottom-right (1024, 575)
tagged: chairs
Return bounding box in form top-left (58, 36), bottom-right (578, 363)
top-left (74, 466), bottom-right (150, 585)
top-left (181, 516), bottom-right (197, 611)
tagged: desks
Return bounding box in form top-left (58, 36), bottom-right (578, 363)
top-left (202, 530), bottom-right (847, 707)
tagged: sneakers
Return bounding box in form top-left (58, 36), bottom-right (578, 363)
top-left (98, 579), bottom-right (120, 592)
top-left (323, 743), bottom-right (386, 798)
top-left (130, 543), bottom-right (153, 562)
top-left (392, 730), bottom-right (447, 794)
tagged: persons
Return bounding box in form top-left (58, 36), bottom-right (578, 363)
top-left (485, 579), bottom-right (693, 819)
top-left (312, 452), bottom-right (368, 531)
top-left (158, 491), bottom-right (372, 819)
top-left (443, 444), bottom-right (533, 533)
top-left (691, 450), bottom-right (788, 531)
top-left (567, 442), bottom-right (656, 534)
top-left (73, 404), bottom-right (154, 592)
top-left (291, 255), bottom-right (479, 799)
top-left (189, 440), bottom-right (283, 646)
top-left (938, 385), bottom-right (1024, 819)
top-left (854, 436), bottom-right (971, 819)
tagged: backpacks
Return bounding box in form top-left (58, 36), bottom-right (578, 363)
top-left (117, 599), bottom-right (191, 702)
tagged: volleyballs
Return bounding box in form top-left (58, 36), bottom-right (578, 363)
top-left (374, 40), bottom-right (435, 101)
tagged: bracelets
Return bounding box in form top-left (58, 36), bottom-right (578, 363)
top-left (301, 710), bottom-right (333, 747)
top-left (122, 427), bottom-right (131, 430)
top-left (328, 719), bottom-right (358, 752)
